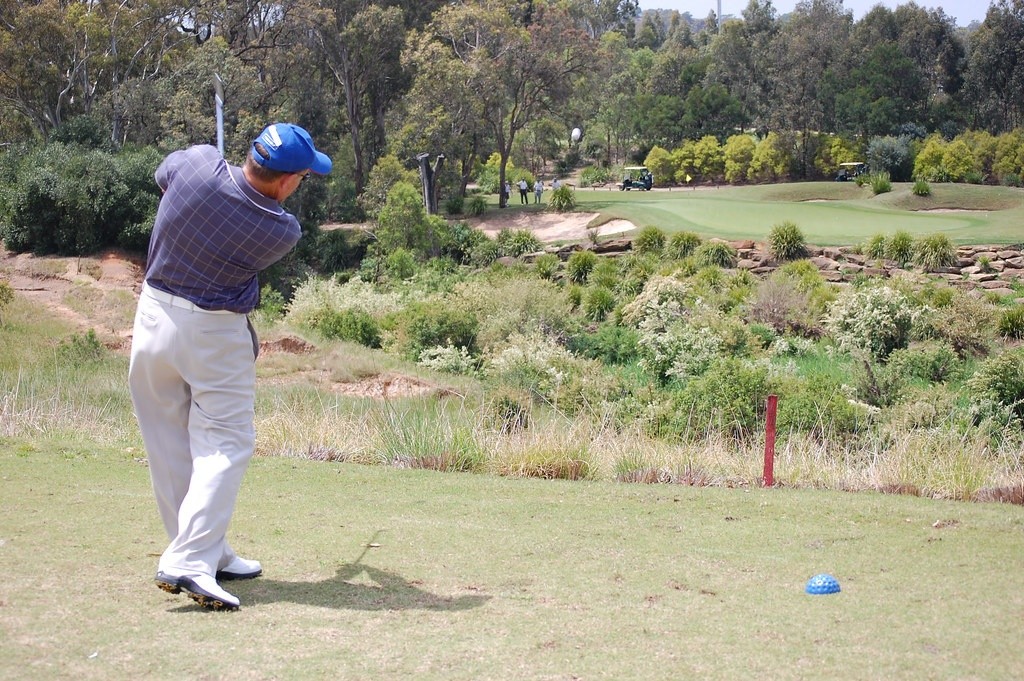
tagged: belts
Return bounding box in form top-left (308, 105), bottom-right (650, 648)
top-left (142, 281), bottom-right (235, 314)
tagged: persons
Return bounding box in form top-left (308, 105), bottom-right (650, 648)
top-left (505, 181), bottom-right (510, 205)
top-left (552, 178), bottom-right (559, 191)
top-left (516, 177), bottom-right (528, 205)
top-left (640, 174), bottom-right (645, 182)
top-left (128, 124), bottom-right (332, 612)
top-left (852, 170), bottom-right (859, 177)
top-left (534, 179), bottom-right (543, 204)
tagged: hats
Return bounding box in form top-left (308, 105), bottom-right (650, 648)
top-left (252, 123), bottom-right (333, 175)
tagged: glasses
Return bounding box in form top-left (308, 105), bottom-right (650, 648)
top-left (293, 171), bottom-right (310, 182)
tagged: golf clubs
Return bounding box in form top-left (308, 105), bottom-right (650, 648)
top-left (214, 73), bottom-right (225, 157)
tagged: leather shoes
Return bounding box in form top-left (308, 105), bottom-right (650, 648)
top-left (216, 557), bottom-right (263, 580)
top-left (156, 566), bottom-right (240, 611)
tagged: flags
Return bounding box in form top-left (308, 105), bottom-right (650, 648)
top-left (686, 174), bottom-right (692, 182)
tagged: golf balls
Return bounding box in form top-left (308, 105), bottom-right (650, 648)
top-left (571, 127), bottom-right (581, 141)
